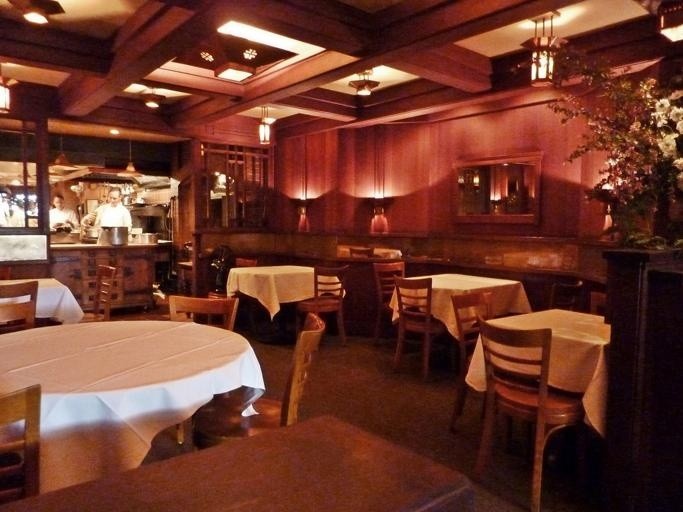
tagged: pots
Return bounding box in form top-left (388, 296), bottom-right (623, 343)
top-left (82, 226), bottom-right (101, 239)
top-left (102, 226), bottom-right (128, 247)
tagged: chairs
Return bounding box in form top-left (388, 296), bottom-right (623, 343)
top-left (548, 276), bottom-right (585, 311)
top-left (1, 380), bottom-right (43, 500)
top-left (169, 291), bottom-right (240, 334)
top-left (475, 314), bottom-right (587, 512)
top-left (373, 261), bottom-right (407, 348)
top-left (394, 274), bottom-right (442, 381)
top-left (191, 311), bottom-right (327, 450)
top-left (451, 291), bottom-right (494, 427)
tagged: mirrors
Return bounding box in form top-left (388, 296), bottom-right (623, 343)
top-left (450, 151), bottom-right (542, 226)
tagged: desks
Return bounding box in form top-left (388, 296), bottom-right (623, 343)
top-left (1, 409), bottom-right (473, 510)
top-left (464, 308), bottom-right (611, 438)
top-left (0, 317), bottom-right (268, 486)
top-left (386, 273), bottom-right (534, 373)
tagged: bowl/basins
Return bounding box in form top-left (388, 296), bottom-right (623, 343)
top-left (143, 232), bottom-right (159, 245)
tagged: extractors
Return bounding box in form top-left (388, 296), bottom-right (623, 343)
top-left (57, 168), bottom-right (172, 190)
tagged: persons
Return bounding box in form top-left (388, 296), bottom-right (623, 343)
top-left (49, 193), bottom-right (79, 231)
top-left (0, 193), bottom-right (10, 227)
top-left (80, 187), bottom-right (133, 235)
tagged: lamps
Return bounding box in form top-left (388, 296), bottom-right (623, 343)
top-left (116, 141), bottom-right (143, 180)
top-left (256, 108), bottom-right (271, 145)
top-left (345, 71), bottom-right (379, 101)
top-left (1, 83), bottom-right (13, 114)
top-left (49, 135), bottom-right (79, 170)
top-left (523, 13), bottom-right (565, 87)
